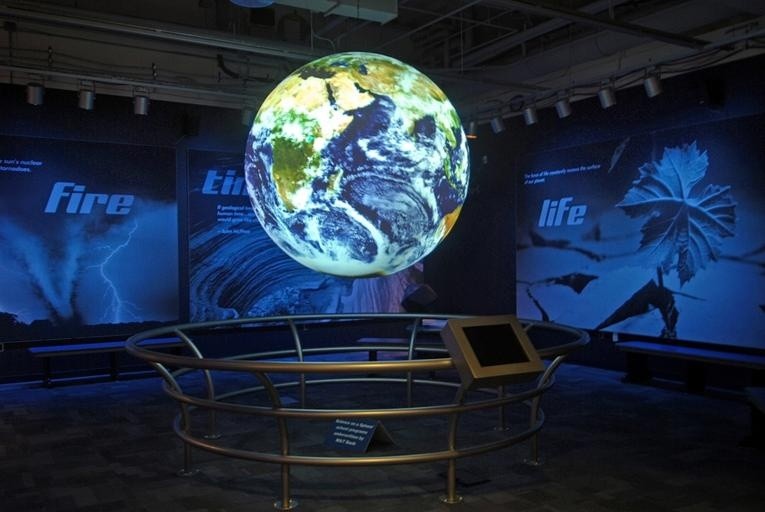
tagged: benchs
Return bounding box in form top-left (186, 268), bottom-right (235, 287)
top-left (353, 336), bottom-right (462, 380)
top-left (26, 334), bottom-right (196, 391)
top-left (612, 336), bottom-right (765, 400)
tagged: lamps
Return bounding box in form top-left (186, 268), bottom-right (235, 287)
top-left (19, 77), bottom-right (154, 121)
top-left (464, 69), bottom-right (665, 143)
top-left (238, 98), bottom-right (260, 134)
